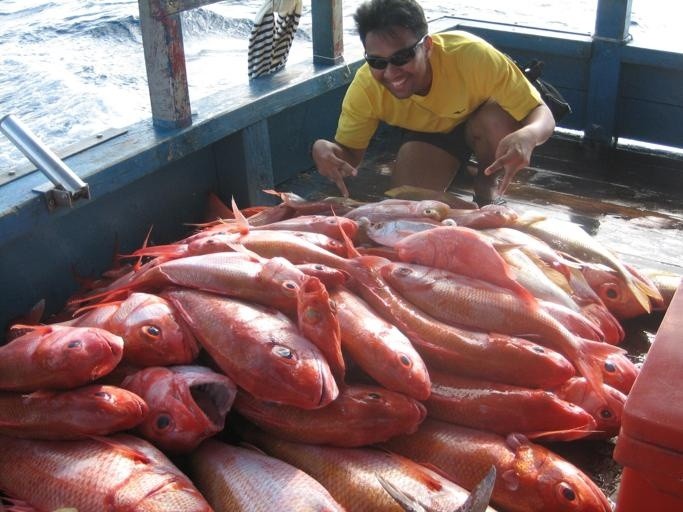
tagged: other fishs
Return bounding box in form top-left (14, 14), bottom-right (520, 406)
top-left (0, 188), bottom-right (669, 511)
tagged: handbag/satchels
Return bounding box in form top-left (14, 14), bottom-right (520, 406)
top-left (507, 54), bottom-right (571, 122)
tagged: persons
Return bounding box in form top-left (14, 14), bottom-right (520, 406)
top-left (313, 0), bottom-right (557, 207)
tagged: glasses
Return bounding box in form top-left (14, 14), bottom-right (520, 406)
top-left (363, 34), bottom-right (428, 69)
